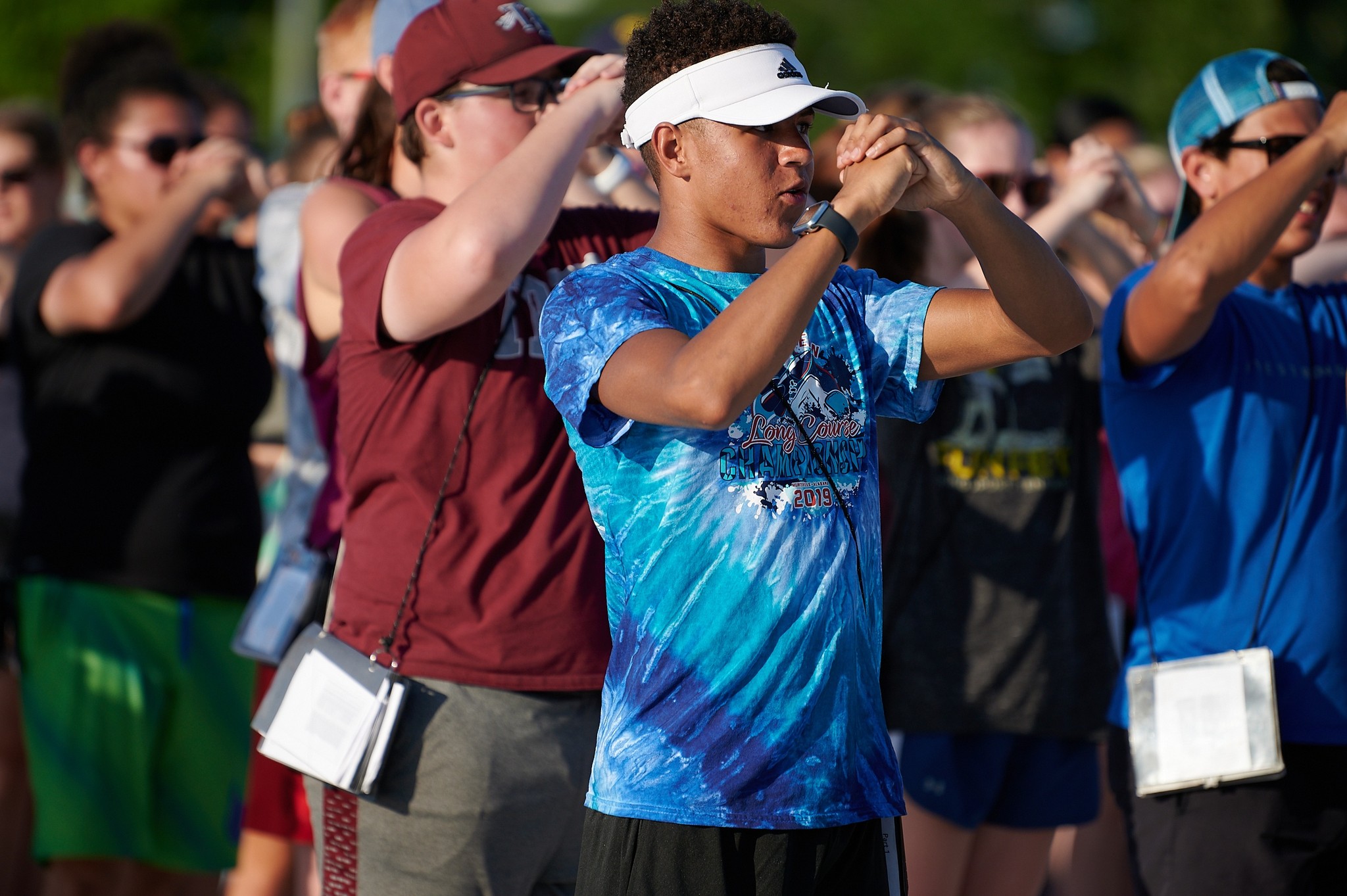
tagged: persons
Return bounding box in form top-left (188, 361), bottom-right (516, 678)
top-left (809, 77), bottom-right (1347, 896)
top-left (1101, 43), bottom-right (1347, 896)
top-left (538, 0), bottom-right (1095, 896)
top-left (2, 28), bottom-right (271, 896)
top-left (0, 0), bottom-right (663, 896)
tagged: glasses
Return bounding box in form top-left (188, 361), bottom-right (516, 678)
top-left (1231, 133), bottom-right (1308, 168)
top-left (117, 135), bottom-right (206, 166)
top-left (977, 172), bottom-right (1053, 209)
top-left (443, 73), bottom-right (569, 114)
top-left (3, 168), bottom-right (30, 183)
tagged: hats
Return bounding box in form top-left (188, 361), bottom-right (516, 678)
top-left (621, 41), bottom-right (868, 149)
top-left (370, 0), bottom-right (443, 66)
top-left (391, 1), bottom-right (602, 124)
top-left (1162, 49), bottom-right (1327, 248)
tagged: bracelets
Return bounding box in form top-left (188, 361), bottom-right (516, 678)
top-left (586, 142), bottom-right (636, 194)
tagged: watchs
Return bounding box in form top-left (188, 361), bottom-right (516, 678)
top-left (793, 199), bottom-right (860, 263)
